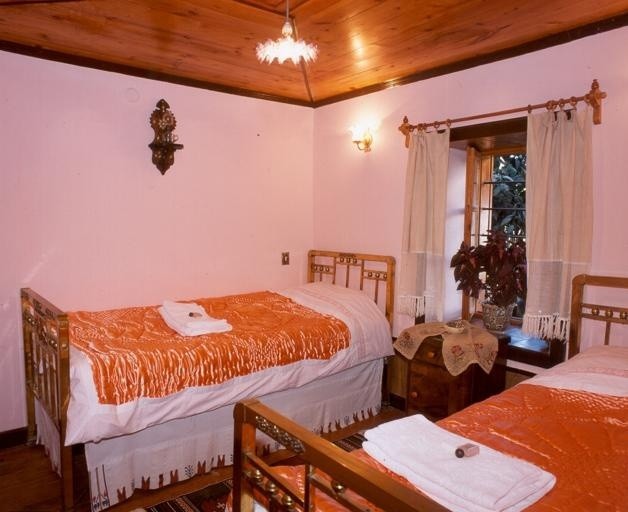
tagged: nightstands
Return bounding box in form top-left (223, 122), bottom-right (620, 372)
top-left (405, 322), bottom-right (512, 421)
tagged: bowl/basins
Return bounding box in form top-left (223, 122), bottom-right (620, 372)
top-left (443, 321), bottom-right (466, 335)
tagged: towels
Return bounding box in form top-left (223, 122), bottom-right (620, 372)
top-left (158, 300), bottom-right (232, 340)
top-left (361, 413), bottom-right (556, 511)
top-left (391, 319), bottom-right (498, 377)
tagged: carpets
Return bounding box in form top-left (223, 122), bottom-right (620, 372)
top-left (132, 422), bottom-right (367, 512)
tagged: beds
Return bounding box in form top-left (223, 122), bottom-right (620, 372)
top-left (19, 251), bottom-right (397, 512)
top-left (232, 275), bottom-right (627, 512)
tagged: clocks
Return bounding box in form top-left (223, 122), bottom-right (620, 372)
top-left (150, 99), bottom-right (182, 176)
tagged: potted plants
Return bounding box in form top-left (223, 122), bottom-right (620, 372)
top-left (449, 228), bottom-right (528, 332)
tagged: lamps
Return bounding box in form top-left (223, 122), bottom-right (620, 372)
top-left (255, 0), bottom-right (321, 67)
top-left (354, 129), bottom-right (373, 151)
